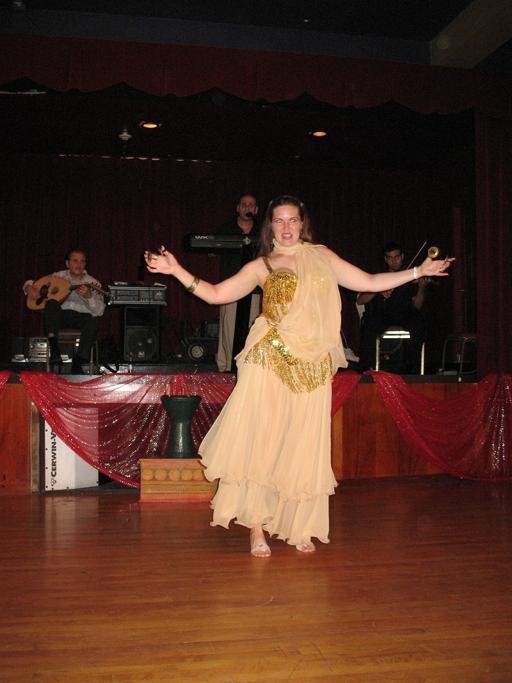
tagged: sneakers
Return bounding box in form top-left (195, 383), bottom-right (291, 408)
top-left (49, 353), bottom-right (84, 375)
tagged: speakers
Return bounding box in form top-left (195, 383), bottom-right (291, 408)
top-left (120, 305), bottom-right (162, 363)
top-left (186, 334), bottom-right (218, 366)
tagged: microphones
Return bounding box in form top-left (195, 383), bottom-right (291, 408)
top-left (246, 211), bottom-right (260, 224)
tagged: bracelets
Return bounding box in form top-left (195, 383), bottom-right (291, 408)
top-left (186, 276), bottom-right (200, 292)
top-left (412, 267), bottom-right (421, 280)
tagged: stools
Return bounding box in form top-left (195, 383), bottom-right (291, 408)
top-left (47, 328), bottom-right (100, 376)
top-left (374, 325), bottom-right (427, 376)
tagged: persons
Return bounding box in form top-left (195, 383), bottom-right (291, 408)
top-left (144, 194), bottom-right (459, 560)
top-left (214, 191), bottom-right (263, 370)
top-left (22, 247), bottom-right (108, 376)
top-left (356, 244), bottom-right (433, 376)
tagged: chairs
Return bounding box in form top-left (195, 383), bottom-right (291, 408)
top-left (437, 286), bottom-right (477, 382)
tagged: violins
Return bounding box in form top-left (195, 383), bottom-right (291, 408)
top-left (398, 261), bottom-right (465, 286)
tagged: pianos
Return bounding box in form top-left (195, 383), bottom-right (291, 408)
top-left (181, 232), bottom-right (260, 254)
